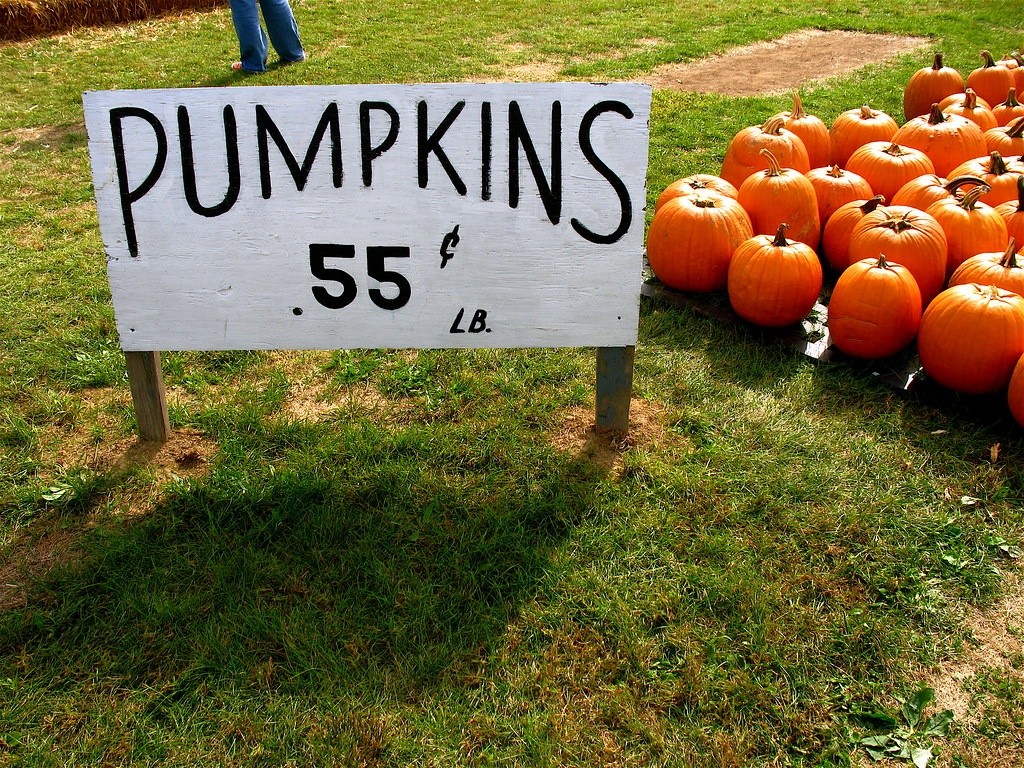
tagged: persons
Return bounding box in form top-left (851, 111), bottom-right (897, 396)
top-left (229, 0), bottom-right (305, 74)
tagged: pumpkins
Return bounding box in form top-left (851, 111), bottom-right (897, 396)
top-left (647, 50), bottom-right (1024, 426)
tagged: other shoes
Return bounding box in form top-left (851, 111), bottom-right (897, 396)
top-left (232, 62), bottom-right (243, 69)
